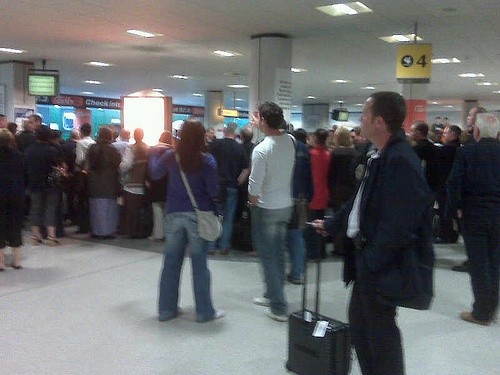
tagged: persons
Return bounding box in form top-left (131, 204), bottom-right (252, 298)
top-left (236, 126), bottom-right (256, 248)
top-left (288, 139), bottom-right (313, 284)
top-left (301, 108), bottom-right (494, 257)
top-left (208, 123), bottom-right (248, 255)
top-left (313, 92), bottom-right (434, 372)
top-left (151, 120), bottom-right (226, 320)
top-left (246, 102), bottom-right (296, 320)
top-left (445, 112), bottom-right (498, 324)
top-left (0, 114), bottom-right (174, 272)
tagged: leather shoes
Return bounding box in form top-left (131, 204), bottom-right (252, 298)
top-left (460, 313), bottom-right (491, 326)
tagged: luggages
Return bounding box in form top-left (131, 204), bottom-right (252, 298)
top-left (285, 221), bottom-right (351, 375)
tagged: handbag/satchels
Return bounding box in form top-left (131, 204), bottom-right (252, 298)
top-left (47, 159), bottom-right (68, 190)
top-left (174, 149), bottom-right (224, 243)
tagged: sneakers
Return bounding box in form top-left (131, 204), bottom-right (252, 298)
top-left (252, 297), bottom-right (272, 307)
top-left (265, 309), bottom-right (288, 322)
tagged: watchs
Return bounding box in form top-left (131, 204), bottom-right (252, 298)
top-left (244, 201), bottom-right (254, 207)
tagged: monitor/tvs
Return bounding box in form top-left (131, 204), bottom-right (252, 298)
top-left (27, 67), bottom-right (59, 97)
top-left (338, 110), bottom-right (349, 122)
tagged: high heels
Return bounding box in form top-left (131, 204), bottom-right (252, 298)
top-left (31, 235), bottom-right (44, 245)
top-left (46, 236), bottom-right (63, 246)
